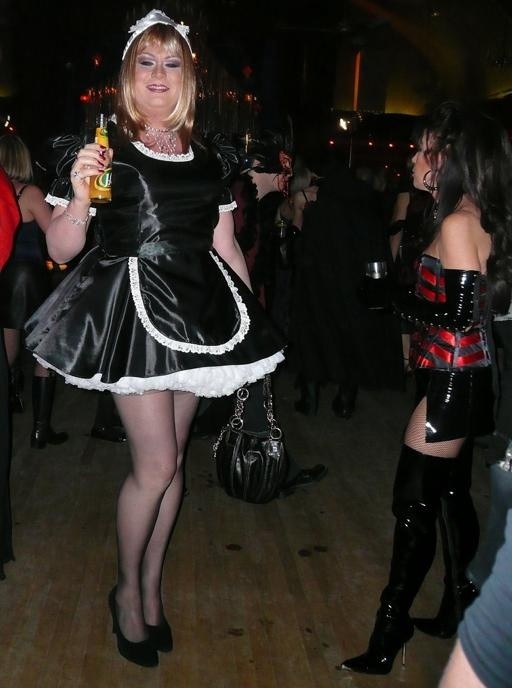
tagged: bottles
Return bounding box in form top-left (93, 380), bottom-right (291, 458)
top-left (88, 112), bottom-right (113, 203)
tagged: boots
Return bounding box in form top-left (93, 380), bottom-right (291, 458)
top-left (8, 361), bottom-right (24, 412)
top-left (413, 436), bottom-right (499, 639)
top-left (341, 444), bottom-right (455, 675)
top-left (30, 375), bottom-right (69, 448)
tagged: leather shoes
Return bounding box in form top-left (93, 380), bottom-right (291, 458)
top-left (91, 416), bottom-right (125, 442)
top-left (293, 393), bottom-right (318, 417)
top-left (332, 394), bottom-right (352, 416)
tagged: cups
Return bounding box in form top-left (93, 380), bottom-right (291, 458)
top-left (366, 261), bottom-right (387, 310)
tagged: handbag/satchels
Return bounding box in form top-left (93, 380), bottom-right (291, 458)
top-left (216, 424), bottom-right (284, 502)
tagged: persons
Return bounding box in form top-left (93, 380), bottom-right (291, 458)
top-left (437, 509), bottom-right (512, 688)
top-left (338, 100), bottom-right (512, 675)
top-left (24, 7), bottom-right (289, 667)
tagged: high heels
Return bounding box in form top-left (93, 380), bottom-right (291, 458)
top-left (143, 591), bottom-right (173, 651)
top-left (108, 585), bottom-right (159, 667)
